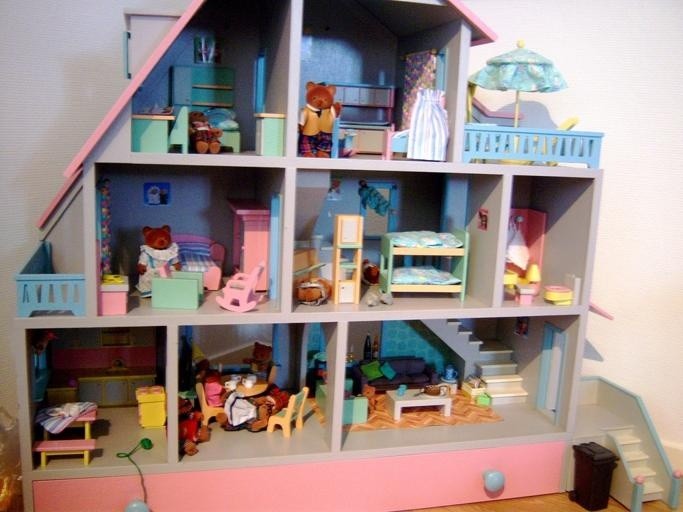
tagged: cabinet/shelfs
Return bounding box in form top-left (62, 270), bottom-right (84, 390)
top-left (11, 1), bottom-right (605, 511)
